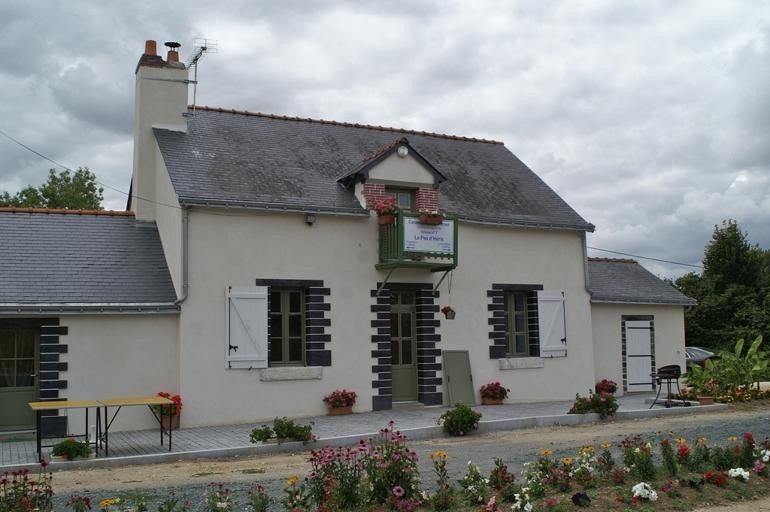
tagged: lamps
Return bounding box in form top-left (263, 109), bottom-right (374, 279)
top-left (305, 214), bottom-right (316, 226)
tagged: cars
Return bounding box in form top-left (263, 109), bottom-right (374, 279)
top-left (685, 347), bottom-right (719, 372)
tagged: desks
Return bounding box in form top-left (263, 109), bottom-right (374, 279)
top-left (28, 401), bottom-right (102, 461)
top-left (98, 396), bottom-right (174, 457)
top-left (649, 376), bottom-right (686, 410)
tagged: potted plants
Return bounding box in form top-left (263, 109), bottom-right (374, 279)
top-left (249, 416), bottom-right (312, 445)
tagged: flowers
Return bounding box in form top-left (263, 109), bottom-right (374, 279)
top-left (479, 382), bottom-right (510, 401)
top-left (700, 381), bottom-right (717, 397)
top-left (374, 196), bottom-right (401, 217)
top-left (322, 387), bottom-right (358, 409)
top-left (52, 437), bottom-right (92, 459)
top-left (432, 399), bottom-right (483, 435)
top-left (441, 306), bottom-right (450, 314)
top-left (149, 392), bottom-right (182, 417)
top-left (566, 378), bottom-right (619, 421)
top-left (418, 209), bottom-right (447, 219)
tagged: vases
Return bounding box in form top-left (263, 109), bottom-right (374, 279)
top-left (49, 452), bottom-right (96, 462)
top-left (419, 215), bottom-right (443, 224)
top-left (328, 406), bottom-right (353, 415)
top-left (446, 310), bottom-right (455, 319)
top-left (482, 398), bottom-right (503, 405)
top-left (699, 395), bottom-right (713, 405)
top-left (161, 412), bottom-right (177, 430)
top-left (378, 214), bottom-right (394, 224)
